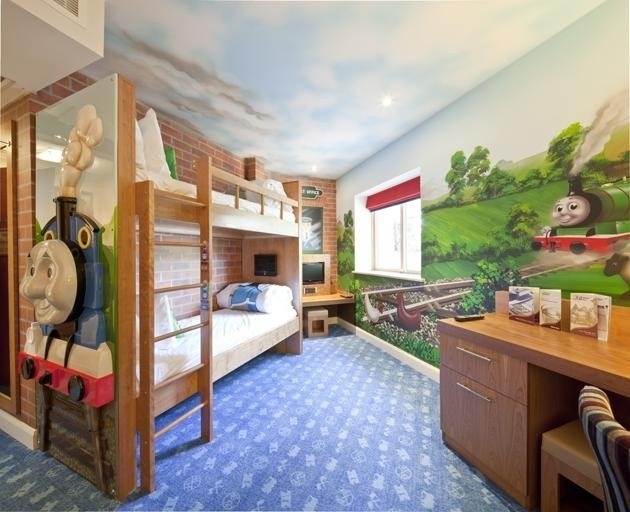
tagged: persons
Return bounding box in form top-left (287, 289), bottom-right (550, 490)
top-left (302, 217), bottom-right (320, 249)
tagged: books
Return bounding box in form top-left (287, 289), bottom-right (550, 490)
top-left (508, 284), bottom-right (612, 342)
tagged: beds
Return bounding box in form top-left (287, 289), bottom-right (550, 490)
top-left (136, 157), bottom-right (303, 493)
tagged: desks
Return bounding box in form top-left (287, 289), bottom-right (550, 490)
top-left (303, 293), bottom-right (354, 307)
top-left (437, 291), bottom-right (630, 512)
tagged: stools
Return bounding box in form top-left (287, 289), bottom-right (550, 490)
top-left (540, 419), bottom-right (605, 512)
top-left (305, 308), bottom-right (329, 338)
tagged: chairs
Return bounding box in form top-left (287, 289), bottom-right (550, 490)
top-left (577, 385), bottom-right (630, 512)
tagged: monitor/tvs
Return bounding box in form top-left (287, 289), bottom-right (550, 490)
top-left (254, 254), bottom-right (276, 276)
top-left (303, 262), bottom-right (324, 284)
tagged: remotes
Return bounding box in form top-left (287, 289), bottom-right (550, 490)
top-left (456, 314), bottom-right (483, 321)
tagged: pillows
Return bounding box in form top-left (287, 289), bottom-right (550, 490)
top-left (135, 117), bottom-right (152, 182)
top-left (154, 295), bottom-right (175, 347)
top-left (139, 108), bottom-right (170, 181)
top-left (173, 314), bottom-right (185, 338)
top-left (165, 146), bottom-right (180, 179)
